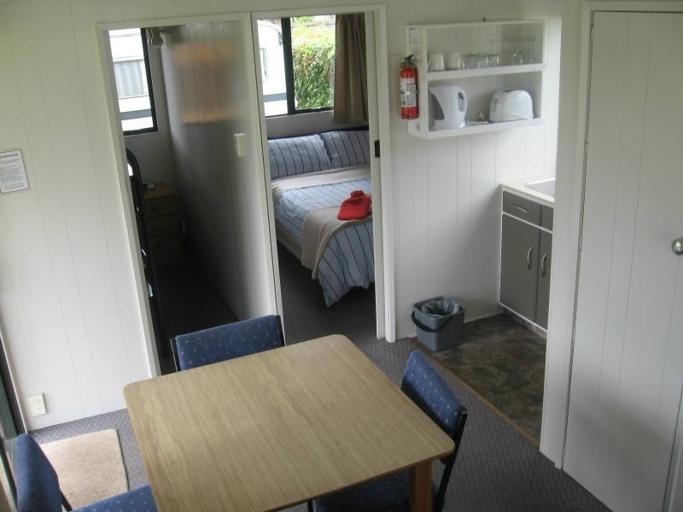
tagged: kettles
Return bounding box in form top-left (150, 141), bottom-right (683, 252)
top-left (428, 86), bottom-right (467, 129)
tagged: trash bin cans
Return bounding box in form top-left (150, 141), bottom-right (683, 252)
top-left (411, 296), bottom-right (466, 351)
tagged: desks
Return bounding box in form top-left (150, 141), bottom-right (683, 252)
top-left (124, 335), bottom-right (455, 512)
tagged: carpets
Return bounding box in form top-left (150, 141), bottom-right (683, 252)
top-left (145, 257), bottom-right (241, 374)
top-left (30, 408), bottom-right (148, 491)
top-left (276, 217), bottom-right (616, 512)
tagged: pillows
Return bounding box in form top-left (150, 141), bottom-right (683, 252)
top-left (266, 134), bottom-right (332, 180)
top-left (321, 131), bottom-right (370, 168)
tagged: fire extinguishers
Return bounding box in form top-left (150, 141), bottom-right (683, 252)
top-left (399, 54), bottom-right (418, 119)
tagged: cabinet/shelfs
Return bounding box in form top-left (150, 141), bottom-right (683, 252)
top-left (498, 178), bottom-right (555, 339)
top-left (143, 182), bottom-right (176, 243)
top-left (404, 20), bottom-right (549, 139)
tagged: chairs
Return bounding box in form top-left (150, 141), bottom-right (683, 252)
top-left (170, 315), bottom-right (313, 512)
top-left (400, 347), bottom-right (469, 512)
top-left (15, 434), bottom-right (157, 512)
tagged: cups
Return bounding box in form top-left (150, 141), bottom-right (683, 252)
top-left (428, 53), bottom-right (444, 71)
top-left (447, 52), bottom-right (464, 68)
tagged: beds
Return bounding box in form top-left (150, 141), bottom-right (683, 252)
top-left (267, 125), bottom-right (375, 308)
